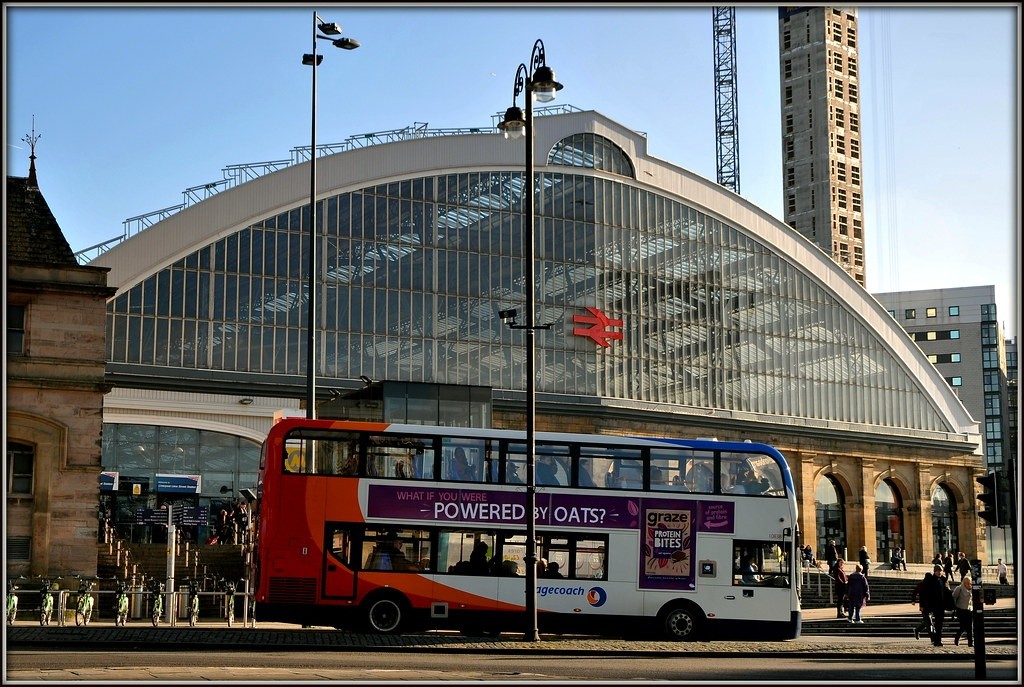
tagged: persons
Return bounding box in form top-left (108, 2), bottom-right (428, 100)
top-left (911, 565), bottom-right (974, 647)
top-left (578, 460), bottom-right (597, 487)
top-left (823, 539), bottom-right (838, 573)
top-left (731, 454), bottom-right (770, 495)
top-left (452, 448), bottom-right (472, 480)
top-left (934, 553), bottom-right (942, 565)
top-left (383, 538), bottom-right (518, 578)
top-left (859, 546), bottom-right (870, 576)
top-left (650, 465), bottom-right (663, 484)
top-left (832, 558), bottom-right (870, 624)
top-left (537, 558), bottom-right (564, 579)
top-left (537, 455), bottom-right (560, 486)
top-left (996, 558), bottom-right (1009, 585)
top-left (955, 552), bottom-right (971, 582)
top-left (736, 553), bottom-right (761, 583)
top-left (214, 502), bottom-right (250, 544)
top-left (890, 547), bottom-right (907, 571)
top-left (506, 463), bottom-right (523, 484)
top-left (800, 544), bottom-right (817, 566)
top-left (943, 553), bottom-right (954, 581)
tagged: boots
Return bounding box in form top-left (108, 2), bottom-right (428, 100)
top-left (837, 611), bottom-right (846, 618)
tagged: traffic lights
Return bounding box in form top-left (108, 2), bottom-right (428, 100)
top-left (974, 473), bottom-right (1009, 527)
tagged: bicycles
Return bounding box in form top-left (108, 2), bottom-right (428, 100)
top-left (7, 573), bottom-right (247, 627)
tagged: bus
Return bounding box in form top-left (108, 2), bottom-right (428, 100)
top-left (253, 416), bottom-right (807, 642)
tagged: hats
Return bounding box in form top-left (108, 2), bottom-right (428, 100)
top-left (934, 564), bottom-right (942, 571)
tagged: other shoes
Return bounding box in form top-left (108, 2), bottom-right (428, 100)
top-left (934, 642), bottom-right (943, 646)
top-left (847, 618), bottom-right (854, 623)
top-left (914, 628), bottom-right (918, 640)
top-left (856, 619), bottom-right (864, 624)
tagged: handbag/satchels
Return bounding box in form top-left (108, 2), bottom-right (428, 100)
top-left (865, 559), bottom-right (871, 564)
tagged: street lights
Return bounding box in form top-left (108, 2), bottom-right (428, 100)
top-left (498, 37), bottom-right (564, 638)
top-left (303, 10), bottom-right (362, 475)
